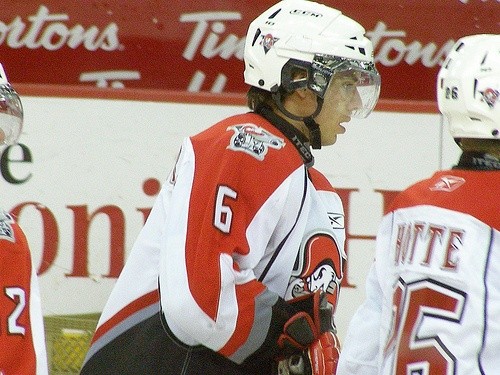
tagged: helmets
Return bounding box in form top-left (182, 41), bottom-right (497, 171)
top-left (437, 33), bottom-right (500, 142)
top-left (244, 0), bottom-right (381, 100)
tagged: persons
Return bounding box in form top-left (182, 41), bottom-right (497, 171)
top-left (337, 33), bottom-right (500, 375)
top-left (80, 0), bottom-right (383, 375)
top-left (0, 62), bottom-right (51, 375)
